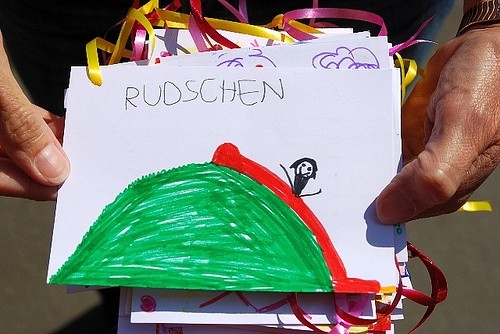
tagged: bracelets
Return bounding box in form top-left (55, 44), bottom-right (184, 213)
top-left (454, 1), bottom-right (500, 39)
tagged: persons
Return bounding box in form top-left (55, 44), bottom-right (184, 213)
top-left (0, 0), bottom-right (500, 227)
